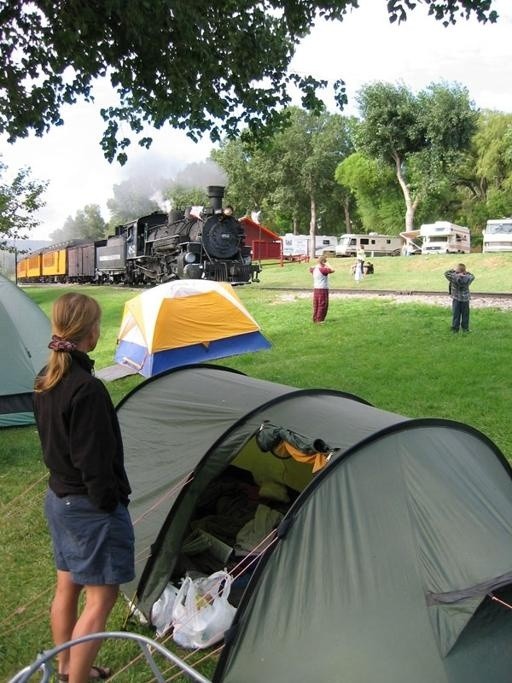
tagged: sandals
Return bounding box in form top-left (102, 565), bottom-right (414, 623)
top-left (57, 663), bottom-right (113, 683)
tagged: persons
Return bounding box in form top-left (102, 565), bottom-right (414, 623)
top-left (354, 255), bottom-right (363, 284)
top-left (444, 263), bottom-right (476, 334)
top-left (356, 244), bottom-right (366, 274)
top-left (308, 256), bottom-right (336, 326)
top-left (29, 291), bottom-right (136, 683)
top-left (363, 261), bottom-right (374, 274)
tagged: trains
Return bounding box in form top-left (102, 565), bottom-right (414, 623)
top-left (17, 186), bottom-right (262, 287)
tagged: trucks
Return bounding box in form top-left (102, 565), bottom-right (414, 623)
top-left (482, 218), bottom-right (512, 253)
top-left (279, 233), bottom-right (404, 257)
top-left (400, 221), bottom-right (470, 255)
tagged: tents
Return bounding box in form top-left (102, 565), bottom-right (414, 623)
top-left (113, 277), bottom-right (274, 380)
top-left (112, 362), bottom-right (511, 683)
top-left (0, 274), bottom-right (58, 428)
